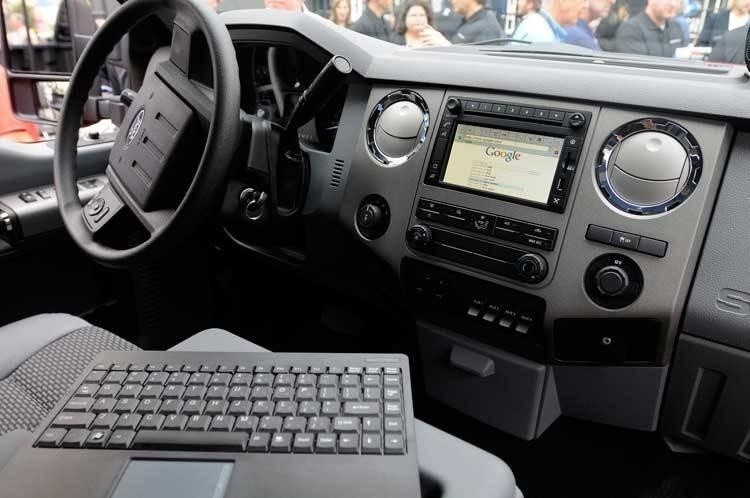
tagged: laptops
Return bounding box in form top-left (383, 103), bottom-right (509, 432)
top-left (1, 349), bottom-right (422, 497)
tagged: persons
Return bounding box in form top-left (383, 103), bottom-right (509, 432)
top-left (4, 10), bottom-right (40, 46)
top-left (209, 0), bottom-right (748, 69)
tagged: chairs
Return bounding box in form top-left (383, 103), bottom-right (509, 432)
top-left (0, 313), bottom-right (166, 497)
